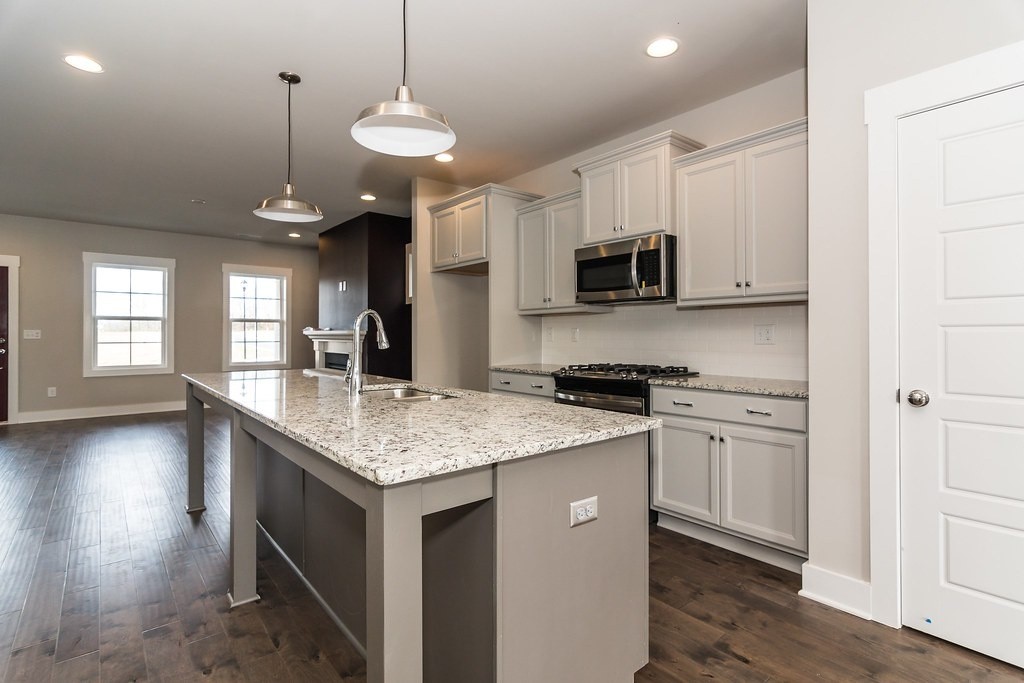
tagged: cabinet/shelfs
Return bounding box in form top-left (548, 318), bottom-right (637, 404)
top-left (570, 127), bottom-right (706, 247)
top-left (671, 116), bottom-right (808, 307)
top-left (515, 187), bottom-right (613, 317)
top-left (427, 182), bottom-right (546, 366)
top-left (488, 370), bottom-right (556, 403)
top-left (318, 210), bottom-right (412, 380)
top-left (650, 386), bottom-right (809, 575)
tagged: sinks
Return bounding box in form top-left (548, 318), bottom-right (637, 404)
top-left (389, 396), bottom-right (460, 402)
top-left (360, 384), bottom-right (432, 400)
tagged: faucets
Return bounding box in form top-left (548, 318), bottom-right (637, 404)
top-left (349, 310), bottom-right (390, 395)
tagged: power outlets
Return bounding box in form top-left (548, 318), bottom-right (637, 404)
top-left (47, 386), bottom-right (57, 398)
top-left (569, 495), bottom-right (598, 527)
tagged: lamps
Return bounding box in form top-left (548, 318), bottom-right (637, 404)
top-left (253, 71), bottom-right (324, 223)
top-left (351, 0), bottom-right (457, 158)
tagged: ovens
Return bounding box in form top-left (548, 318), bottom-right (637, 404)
top-left (554, 390), bottom-right (645, 416)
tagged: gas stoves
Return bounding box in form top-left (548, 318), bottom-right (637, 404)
top-left (552, 364), bottom-right (701, 379)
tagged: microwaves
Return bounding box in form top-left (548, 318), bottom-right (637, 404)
top-left (575, 234), bottom-right (678, 304)
